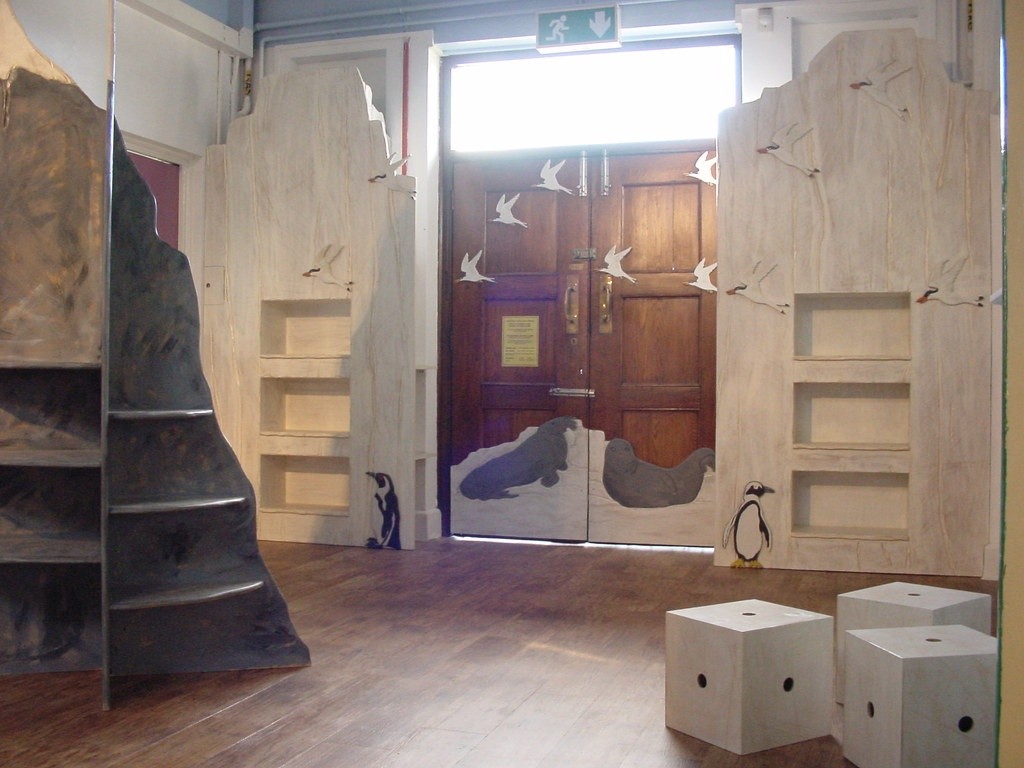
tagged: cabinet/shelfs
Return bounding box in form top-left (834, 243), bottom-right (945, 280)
top-left (715, 27), bottom-right (995, 576)
top-left (222, 60), bottom-right (428, 553)
top-left (1, 65), bottom-right (315, 710)
